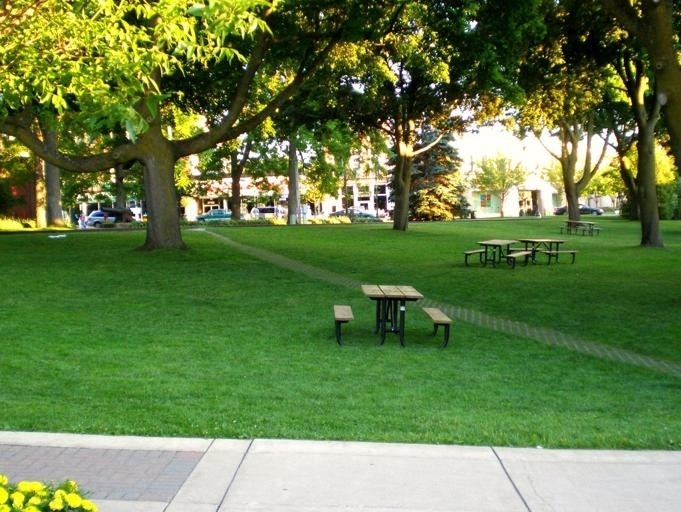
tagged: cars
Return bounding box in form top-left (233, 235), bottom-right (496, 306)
top-left (250, 207), bottom-right (289, 220)
top-left (553, 203), bottom-right (605, 216)
top-left (196, 209), bottom-right (244, 224)
top-left (329, 208), bottom-right (374, 222)
top-left (85, 207), bottom-right (137, 228)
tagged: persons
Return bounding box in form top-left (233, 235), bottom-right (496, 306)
top-left (274, 202), bottom-right (279, 218)
top-left (80, 211), bottom-right (85, 230)
top-left (251, 202), bottom-right (259, 222)
top-left (302, 203), bottom-right (308, 224)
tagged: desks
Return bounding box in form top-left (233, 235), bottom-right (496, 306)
top-left (361, 282), bottom-right (426, 348)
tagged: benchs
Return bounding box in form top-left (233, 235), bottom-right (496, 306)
top-left (424, 305), bottom-right (455, 350)
top-left (459, 216), bottom-right (603, 269)
top-left (331, 303), bottom-right (356, 347)
